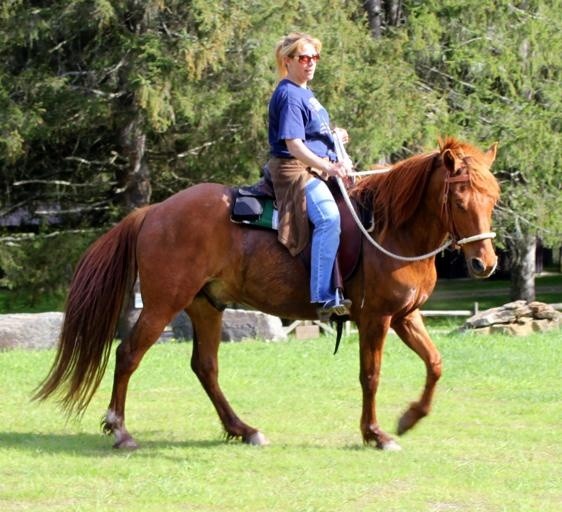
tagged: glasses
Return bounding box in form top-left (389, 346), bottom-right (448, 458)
top-left (288, 53), bottom-right (319, 65)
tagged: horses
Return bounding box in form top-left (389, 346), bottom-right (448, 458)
top-left (27, 133), bottom-right (501, 451)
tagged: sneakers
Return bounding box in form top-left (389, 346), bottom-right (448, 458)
top-left (316, 298), bottom-right (352, 322)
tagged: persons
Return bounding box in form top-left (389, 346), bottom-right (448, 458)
top-left (264, 32), bottom-right (350, 320)
